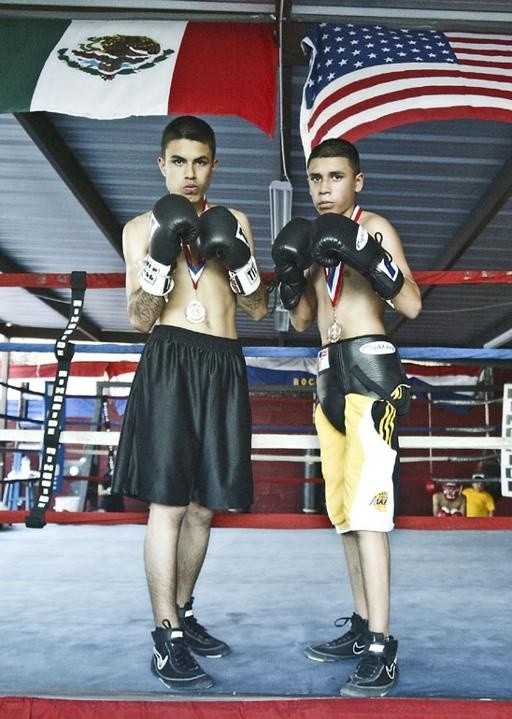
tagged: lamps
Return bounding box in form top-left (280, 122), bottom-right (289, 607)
top-left (268, 180), bottom-right (293, 245)
top-left (274, 285), bottom-right (291, 332)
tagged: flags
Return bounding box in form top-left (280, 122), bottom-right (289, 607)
top-left (1, 18), bottom-right (276, 139)
top-left (298, 20), bottom-right (511, 163)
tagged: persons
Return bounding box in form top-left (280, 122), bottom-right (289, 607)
top-left (432, 472), bottom-right (495, 518)
top-left (272, 140), bottom-right (423, 701)
top-left (97, 451), bottom-right (125, 512)
top-left (113, 116), bottom-right (268, 693)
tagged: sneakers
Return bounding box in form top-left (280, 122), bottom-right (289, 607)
top-left (302, 611), bottom-right (398, 699)
top-left (151, 596), bottom-right (232, 694)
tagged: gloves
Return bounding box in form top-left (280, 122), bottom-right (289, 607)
top-left (436, 506), bottom-right (462, 517)
top-left (271, 212), bottom-right (404, 311)
top-left (140, 194), bottom-right (262, 303)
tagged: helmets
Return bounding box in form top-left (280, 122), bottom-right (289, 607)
top-left (442, 482), bottom-right (463, 500)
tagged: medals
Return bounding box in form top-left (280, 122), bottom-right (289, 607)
top-left (327, 321), bottom-right (343, 343)
top-left (183, 299), bottom-right (206, 323)
